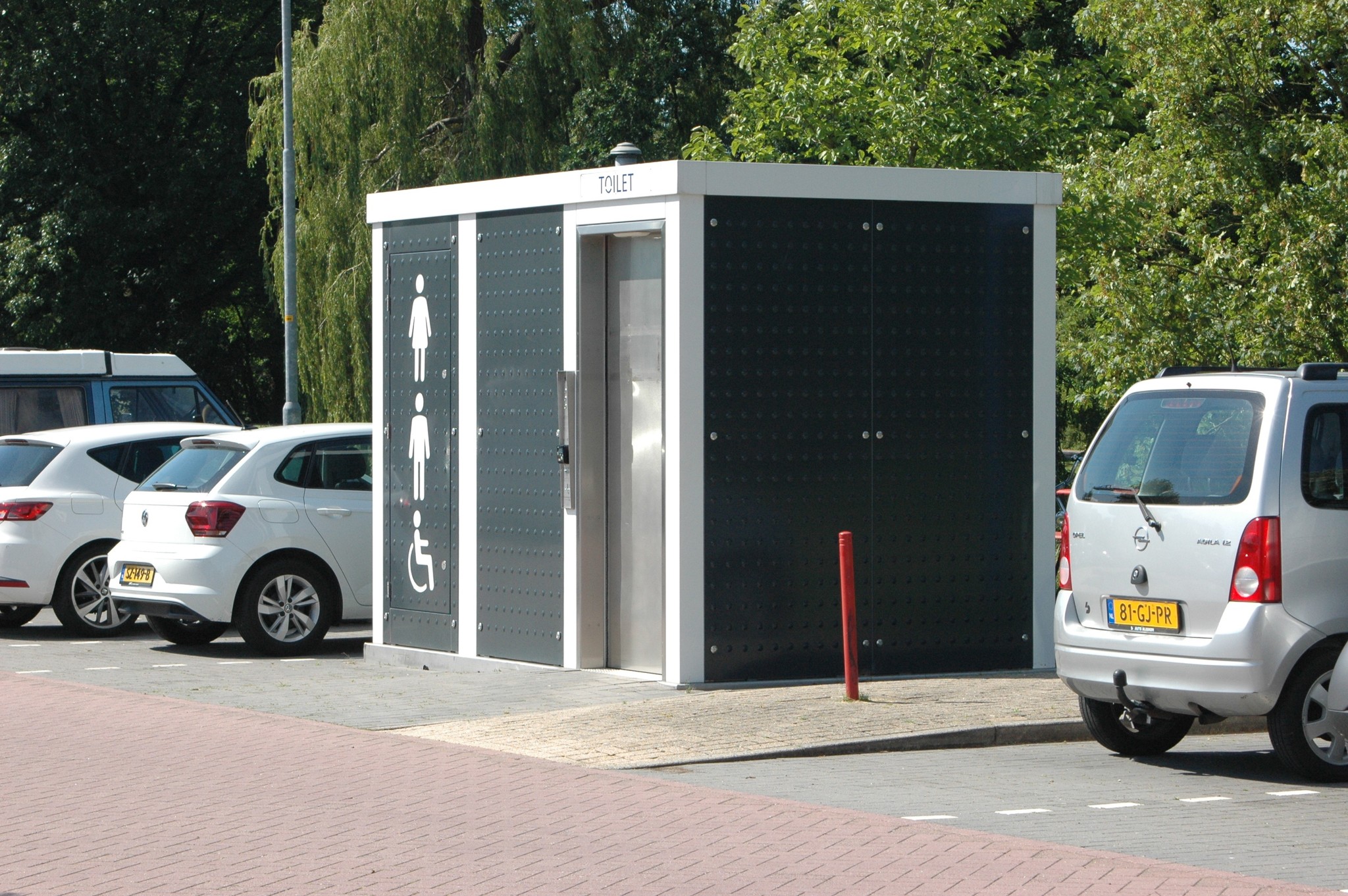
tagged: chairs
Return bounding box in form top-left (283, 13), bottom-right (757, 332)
top-left (1175, 435), bottom-right (1234, 497)
top-left (328, 448), bottom-right (372, 491)
top-left (134, 448), bottom-right (165, 480)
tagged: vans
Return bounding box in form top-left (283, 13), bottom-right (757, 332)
top-left (1, 345), bottom-right (238, 483)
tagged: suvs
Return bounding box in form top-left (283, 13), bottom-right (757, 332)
top-left (105, 421), bottom-right (374, 658)
top-left (1054, 360), bottom-right (1348, 785)
top-left (0, 419), bottom-right (250, 638)
